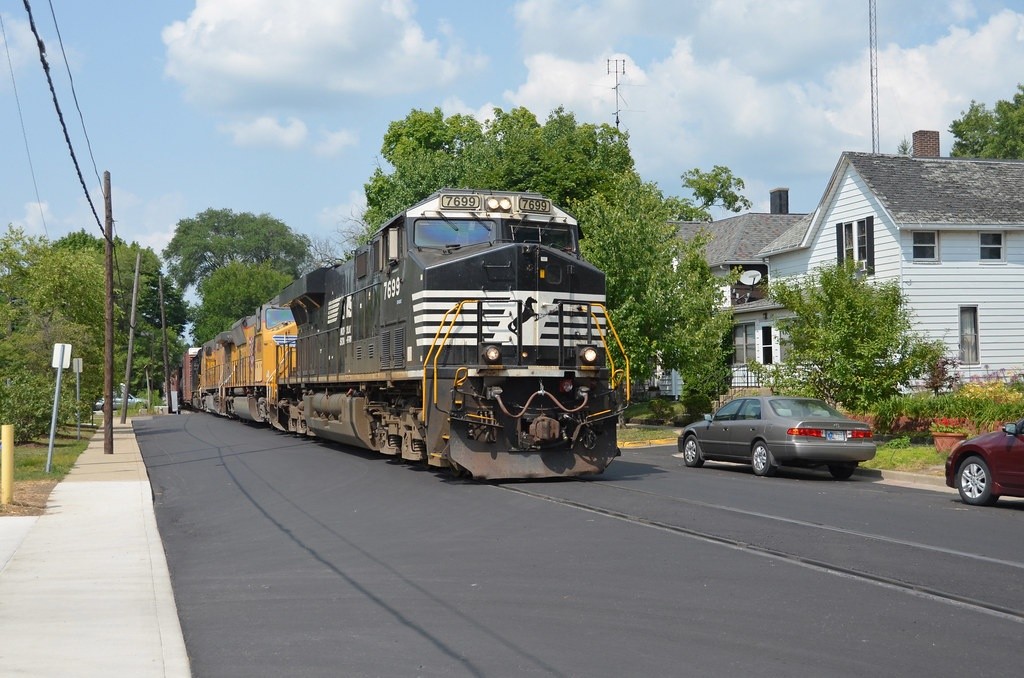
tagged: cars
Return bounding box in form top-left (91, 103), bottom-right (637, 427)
top-left (678, 395), bottom-right (877, 480)
top-left (945, 418), bottom-right (1024, 506)
top-left (94, 389), bottom-right (148, 413)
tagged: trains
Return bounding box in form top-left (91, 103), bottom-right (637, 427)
top-left (162, 185), bottom-right (631, 483)
